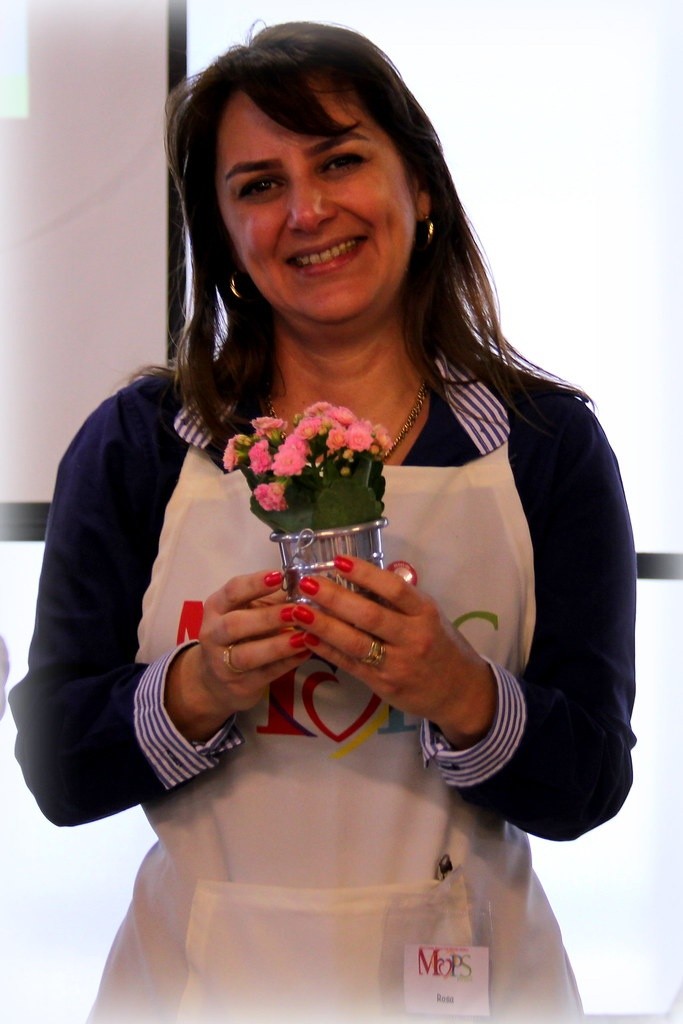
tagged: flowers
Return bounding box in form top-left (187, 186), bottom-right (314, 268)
top-left (223, 402), bottom-right (395, 535)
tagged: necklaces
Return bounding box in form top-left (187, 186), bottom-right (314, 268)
top-left (255, 385), bottom-right (429, 463)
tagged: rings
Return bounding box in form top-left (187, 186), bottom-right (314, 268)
top-left (223, 645), bottom-right (243, 675)
top-left (361, 640), bottom-right (385, 666)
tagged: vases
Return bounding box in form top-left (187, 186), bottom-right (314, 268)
top-left (269, 517), bottom-right (390, 611)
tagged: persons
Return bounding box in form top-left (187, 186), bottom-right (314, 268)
top-left (9, 23), bottom-right (636, 1006)
